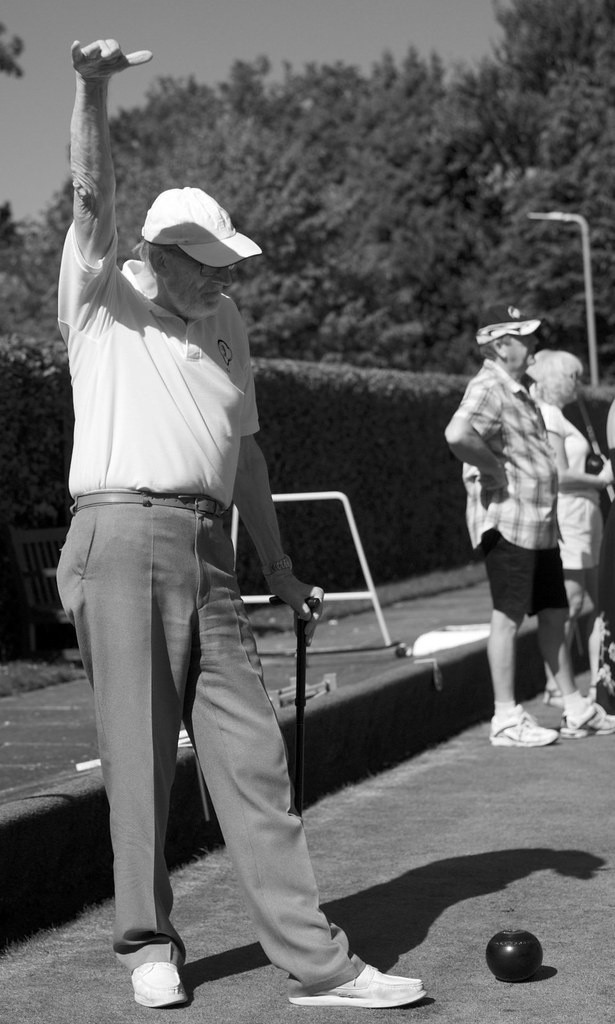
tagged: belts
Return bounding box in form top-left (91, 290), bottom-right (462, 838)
top-left (75, 491), bottom-right (227, 518)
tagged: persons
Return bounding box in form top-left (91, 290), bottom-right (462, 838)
top-left (445, 301), bottom-right (614, 746)
top-left (56, 38), bottom-right (426, 1011)
top-left (523, 348), bottom-right (615, 709)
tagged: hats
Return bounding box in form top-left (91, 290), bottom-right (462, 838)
top-left (141, 186), bottom-right (262, 268)
top-left (474, 305), bottom-right (542, 346)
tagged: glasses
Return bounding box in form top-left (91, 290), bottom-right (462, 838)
top-left (163, 246), bottom-right (235, 276)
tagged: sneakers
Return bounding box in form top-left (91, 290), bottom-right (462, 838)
top-left (489, 704), bottom-right (559, 747)
top-left (560, 695), bottom-right (615, 739)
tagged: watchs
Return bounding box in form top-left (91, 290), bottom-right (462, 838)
top-left (260, 554), bottom-right (293, 576)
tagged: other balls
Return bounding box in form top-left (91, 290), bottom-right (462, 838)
top-left (486, 929), bottom-right (543, 983)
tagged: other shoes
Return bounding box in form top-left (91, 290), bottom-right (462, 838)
top-left (289, 964), bottom-right (428, 1009)
top-left (543, 687), bottom-right (563, 705)
top-left (131, 961), bottom-right (188, 1008)
